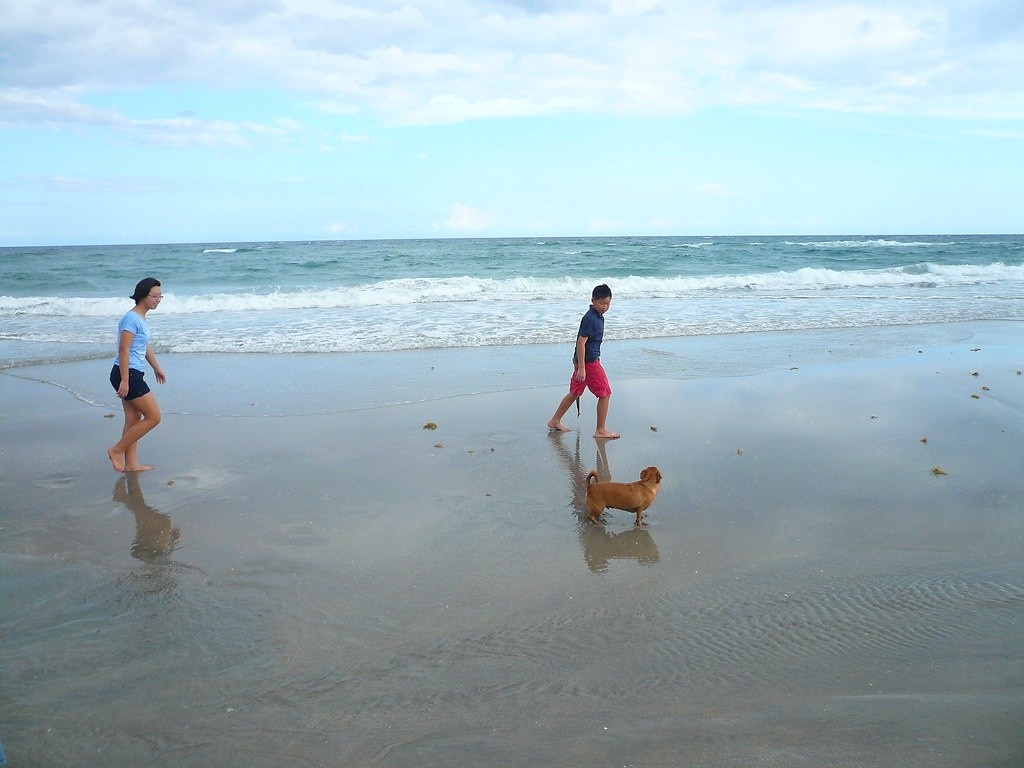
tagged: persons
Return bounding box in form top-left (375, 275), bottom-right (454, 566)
top-left (108, 278), bottom-right (166, 473)
top-left (547, 283), bottom-right (620, 438)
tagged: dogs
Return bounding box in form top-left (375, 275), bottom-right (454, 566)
top-left (585, 466), bottom-right (663, 527)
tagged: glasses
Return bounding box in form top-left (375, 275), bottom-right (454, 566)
top-left (148, 294), bottom-right (164, 300)
